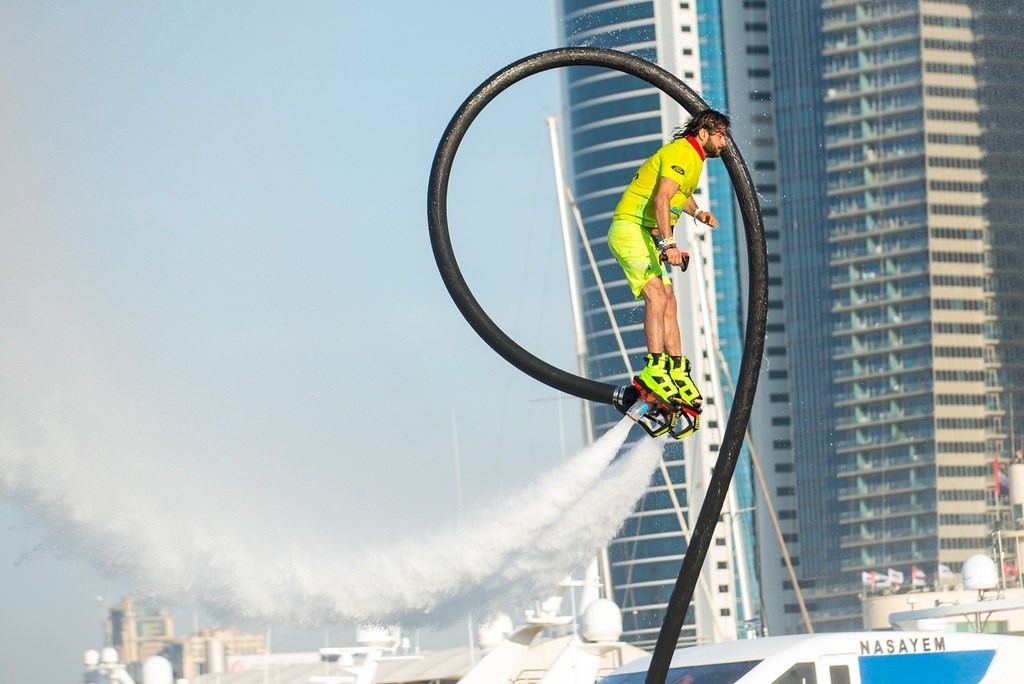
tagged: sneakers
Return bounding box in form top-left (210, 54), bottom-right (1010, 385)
top-left (667, 355), bottom-right (703, 413)
top-left (635, 353), bottom-right (685, 415)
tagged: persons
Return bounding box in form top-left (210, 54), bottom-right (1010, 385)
top-left (608, 109), bottom-right (730, 408)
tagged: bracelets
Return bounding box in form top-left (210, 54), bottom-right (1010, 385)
top-left (692, 208), bottom-right (702, 224)
top-left (659, 236), bottom-right (676, 250)
